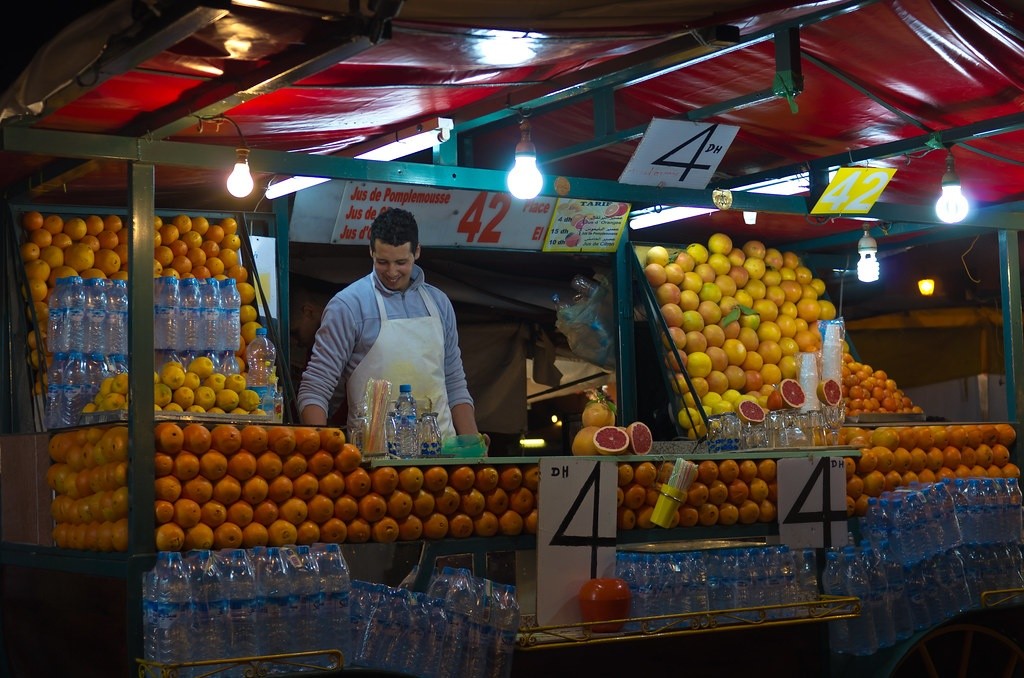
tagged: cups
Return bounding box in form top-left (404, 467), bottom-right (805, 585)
top-left (652, 485), bottom-right (685, 530)
top-left (708, 325), bottom-right (845, 453)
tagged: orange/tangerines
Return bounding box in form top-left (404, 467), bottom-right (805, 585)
top-left (645, 234), bottom-right (924, 436)
top-left (812, 423), bottom-right (1020, 515)
top-left (146, 423), bottom-right (538, 550)
top-left (572, 401), bottom-right (653, 456)
top-left (47, 426), bottom-right (131, 553)
top-left (21, 211), bottom-right (128, 395)
top-left (617, 459), bottom-right (779, 530)
top-left (152, 213), bottom-right (277, 385)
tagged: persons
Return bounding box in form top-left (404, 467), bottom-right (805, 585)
top-left (297, 208), bottom-right (490, 459)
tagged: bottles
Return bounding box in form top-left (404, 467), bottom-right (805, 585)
top-left (246, 328), bottom-right (276, 418)
top-left (551, 274), bottom-right (616, 370)
top-left (44, 276), bottom-right (240, 426)
top-left (615, 478), bottom-right (1024, 634)
top-left (385, 384), bottom-right (443, 459)
top-left (142, 543), bottom-right (521, 677)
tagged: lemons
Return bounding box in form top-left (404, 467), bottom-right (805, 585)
top-left (82, 357), bottom-right (266, 416)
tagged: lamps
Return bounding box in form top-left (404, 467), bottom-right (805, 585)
top-left (260, 116), bottom-right (454, 199)
